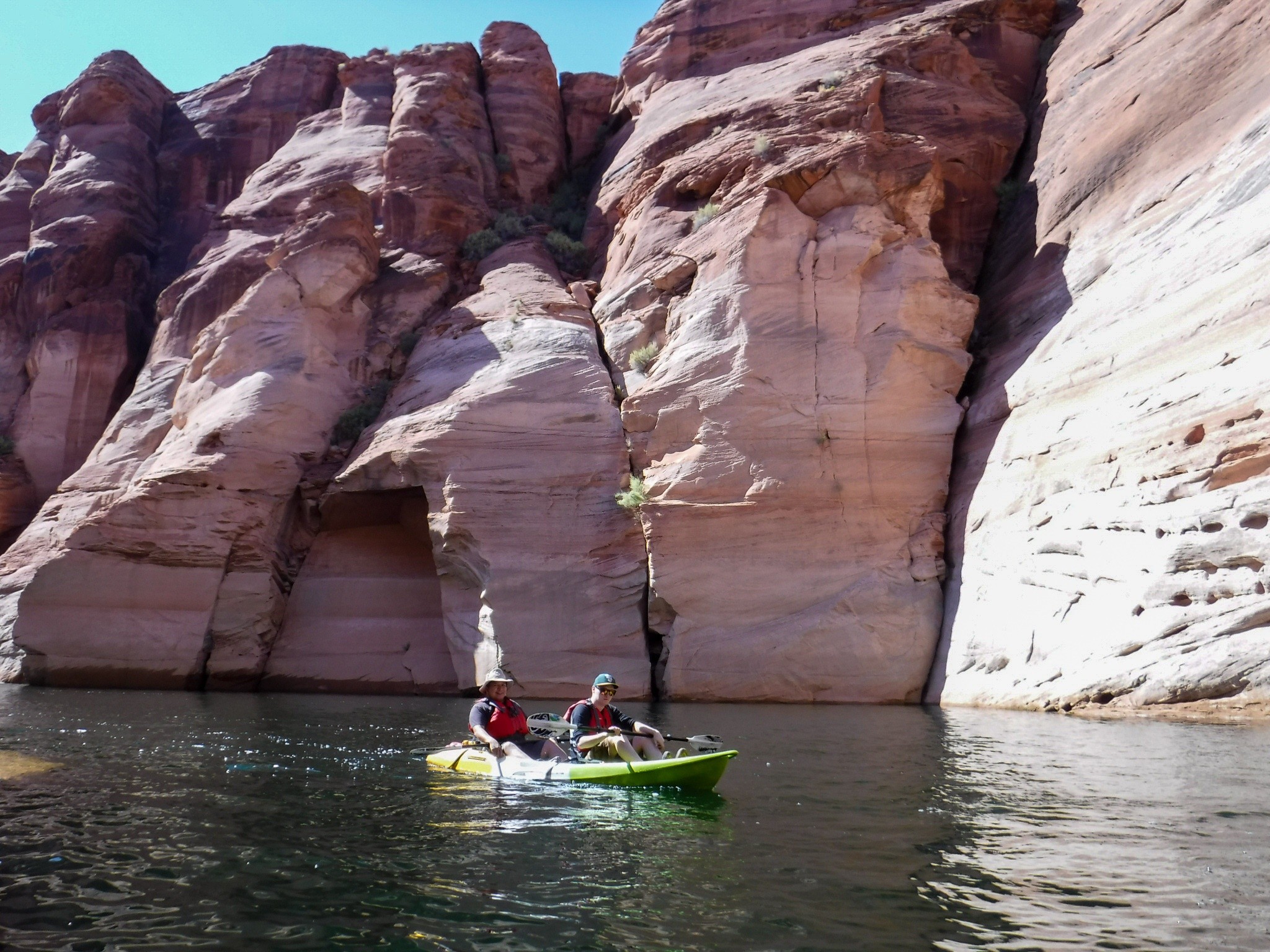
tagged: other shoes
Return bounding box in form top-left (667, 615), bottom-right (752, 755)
top-left (675, 748), bottom-right (689, 759)
top-left (661, 751), bottom-right (674, 761)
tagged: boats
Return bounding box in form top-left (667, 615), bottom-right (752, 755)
top-left (425, 741), bottom-right (739, 791)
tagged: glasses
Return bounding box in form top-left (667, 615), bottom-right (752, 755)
top-left (596, 686), bottom-right (617, 696)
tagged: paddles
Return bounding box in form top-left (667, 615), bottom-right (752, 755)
top-left (410, 737), bottom-right (570, 757)
top-left (526, 712), bottom-right (723, 754)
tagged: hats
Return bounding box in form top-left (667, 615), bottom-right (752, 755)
top-left (594, 673), bottom-right (619, 689)
top-left (479, 668), bottom-right (514, 694)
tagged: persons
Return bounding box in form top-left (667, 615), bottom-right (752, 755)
top-left (569, 673), bottom-right (665, 762)
top-left (469, 668), bottom-right (569, 762)
